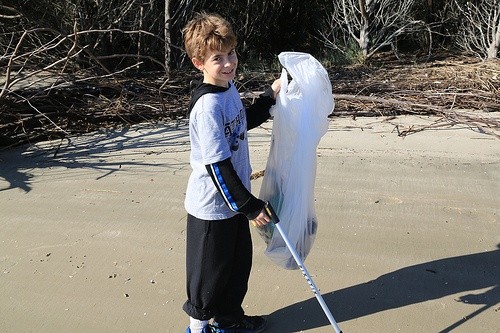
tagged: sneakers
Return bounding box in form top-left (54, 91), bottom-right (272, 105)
top-left (212, 315), bottom-right (268, 333)
top-left (185, 323), bottom-right (224, 333)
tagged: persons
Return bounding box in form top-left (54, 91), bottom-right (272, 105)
top-left (183, 12), bottom-right (282, 333)
